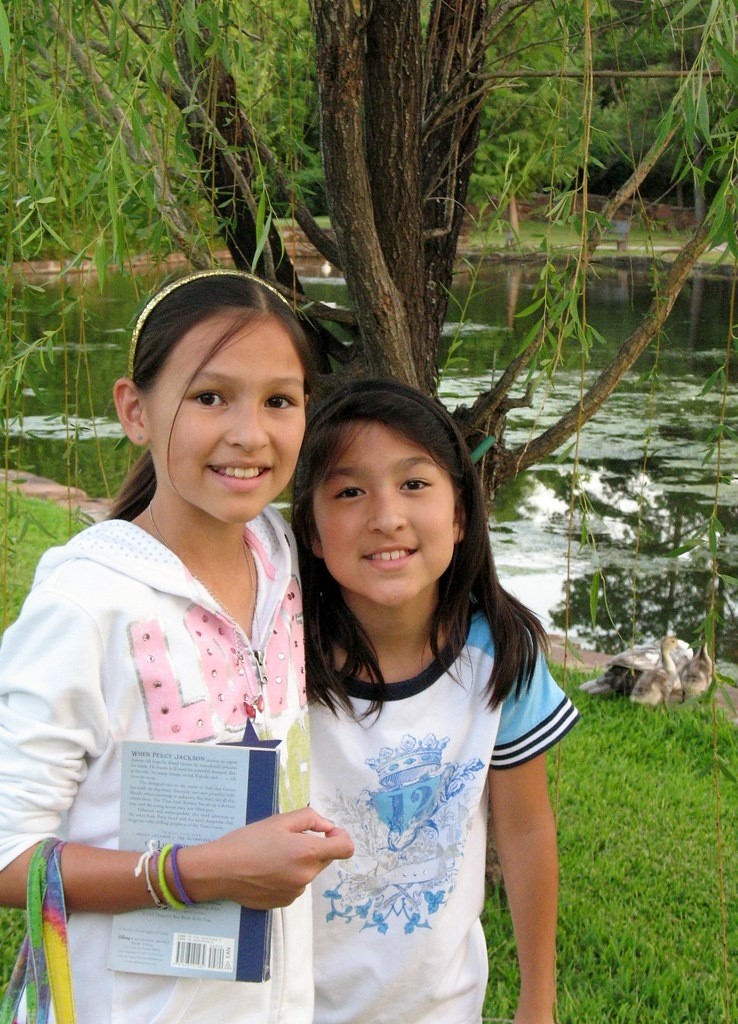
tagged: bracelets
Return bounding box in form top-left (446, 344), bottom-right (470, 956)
top-left (158, 843), bottom-right (188, 912)
top-left (170, 841), bottom-right (200, 906)
top-left (135, 837), bottom-right (171, 911)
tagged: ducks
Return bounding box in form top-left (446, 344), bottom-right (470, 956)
top-left (577, 632), bottom-right (712, 710)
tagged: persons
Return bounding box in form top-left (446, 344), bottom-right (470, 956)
top-left (288, 378), bottom-right (578, 1022)
top-left (0, 272), bottom-right (354, 1024)
